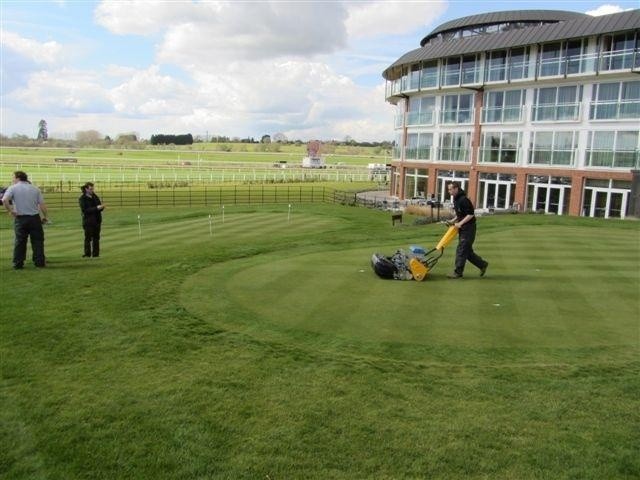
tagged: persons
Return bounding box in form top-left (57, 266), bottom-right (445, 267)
top-left (440, 183), bottom-right (488, 278)
top-left (79, 182), bottom-right (105, 258)
top-left (2, 171), bottom-right (50, 269)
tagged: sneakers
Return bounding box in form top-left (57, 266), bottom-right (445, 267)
top-left (13, 262), bottom-right (46, 269)
top-left (446, 272), bottom-right (463, 278)
top-left (480, 261), bottom-right (489, 278)
top-left (82, 254), bottom-right (99, 258)
